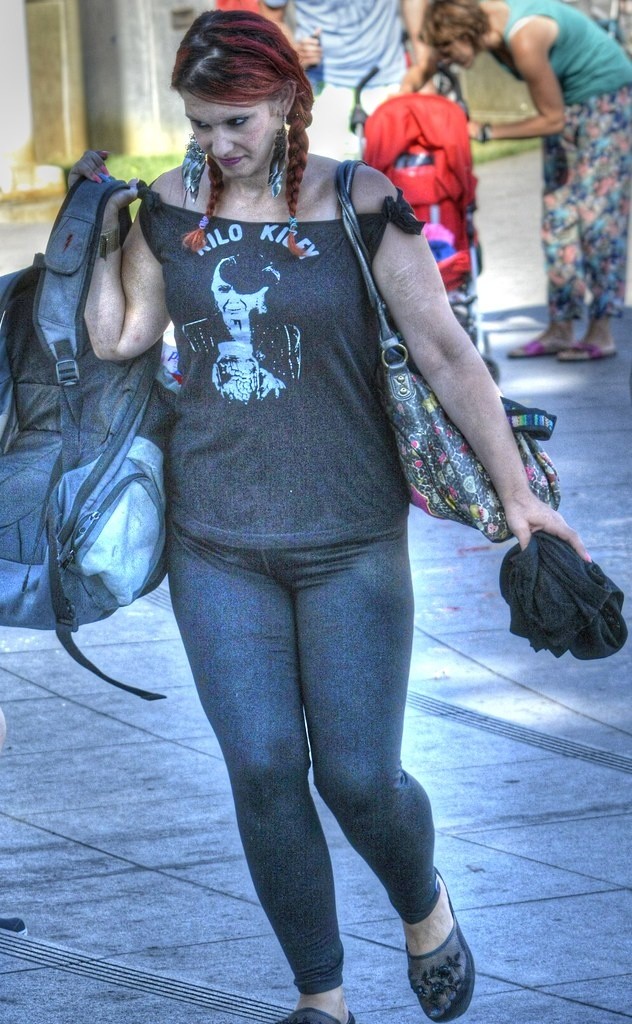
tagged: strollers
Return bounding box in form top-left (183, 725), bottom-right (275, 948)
top-left (351, 61), bottom-right (500, 392)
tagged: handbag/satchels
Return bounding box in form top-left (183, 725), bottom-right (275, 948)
top-left (332, 156), bottom-right (563, 544)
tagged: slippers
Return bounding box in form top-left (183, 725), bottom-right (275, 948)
top-left (556, 341), bottom-right (617, 362)
top-left (506, 338), bottom-right (557, 359)
top-left (274, 1010), bottom-right (355, 1024)
top-left (404, 865), bottom-right (475, 1024)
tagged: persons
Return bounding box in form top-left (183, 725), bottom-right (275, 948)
top-left (0, 710), bottom-right (29, 940)
top-left (260, 0), bottom-right (426, 164)
top-left (399, 0), bottom-right (632, 363)
top-left (63, 10), bottom-right (593, 1024)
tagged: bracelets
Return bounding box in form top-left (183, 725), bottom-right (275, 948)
top-left (477, 122), bottom-right (492, 142)
top-left (95, 225), bottom-right (122, 261)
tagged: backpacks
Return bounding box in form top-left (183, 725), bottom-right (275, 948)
top-left (0, 169), bottom-right (171, 632)
